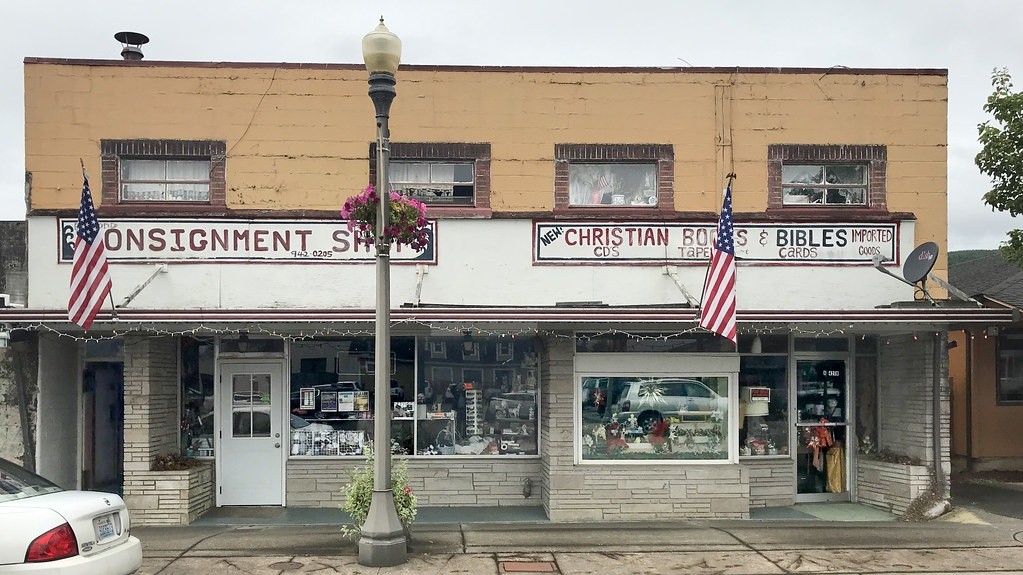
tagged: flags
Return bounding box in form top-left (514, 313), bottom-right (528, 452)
top-left (68, 176), bottom-right (113, 333)
top-left (699, 187), bottom-right (737, 347)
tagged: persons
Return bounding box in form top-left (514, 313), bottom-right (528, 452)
top-left (595, 382), bottom-right (607, 418)
top-left (450, 380), bottom-right (491, 439)
top-left (290, 372), bottom-right (349, 424)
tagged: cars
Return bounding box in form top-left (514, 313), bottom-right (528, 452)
top-left (0, 455), bottom-right (143, 575)
top-left (582, 376), bottom-right (729, 436)
top-left (181, 405), bottom-right (335, 459)
top-left (489, 386), bottom-right (536, 422)
top-left (290, 378), bottom-right (468, 416)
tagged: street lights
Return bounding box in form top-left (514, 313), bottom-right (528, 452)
top-left (357, 14), bottom-right (412, 567)
